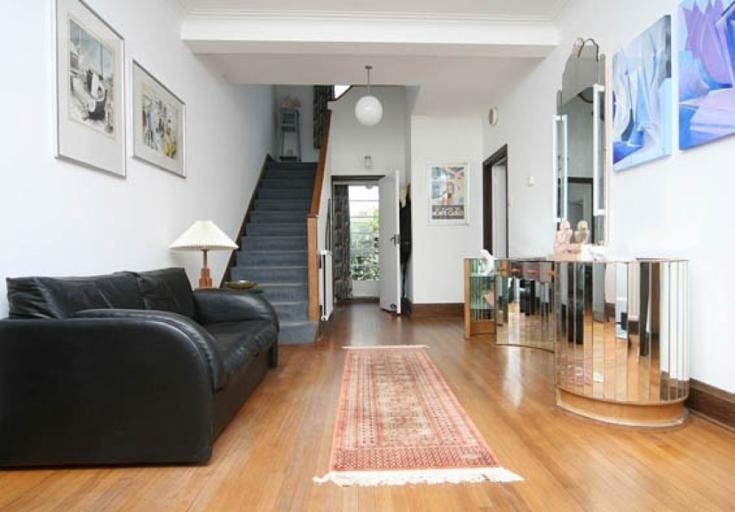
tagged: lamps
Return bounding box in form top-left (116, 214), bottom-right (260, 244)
top-left (363, 155), bottom-right (372, 168)
top-left (354, 64), bottom-right (383, 127)
top-left (168, 221), bottom-right (239, 289)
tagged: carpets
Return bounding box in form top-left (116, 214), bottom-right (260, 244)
top-left (314, 344), bottom-right (524, 485)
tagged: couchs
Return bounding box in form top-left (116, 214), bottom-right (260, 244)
top-left (1, 267), bottom-right (280, 471)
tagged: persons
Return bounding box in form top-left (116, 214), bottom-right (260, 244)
top-left (141, 95), bottom-right (178, 161)
top-left (441, 179), bottom-right (464, 205)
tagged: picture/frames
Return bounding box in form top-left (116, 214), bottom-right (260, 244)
top-left (426, 162), bottom-right (472, 226)
top-left (53, 0), bottom-right (187, 181)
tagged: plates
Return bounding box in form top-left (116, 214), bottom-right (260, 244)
top-left (222, 279), bottom-right (257, 289)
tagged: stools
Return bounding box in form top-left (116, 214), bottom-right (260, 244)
top-left (278, 108), bottom-right (302, 162)
